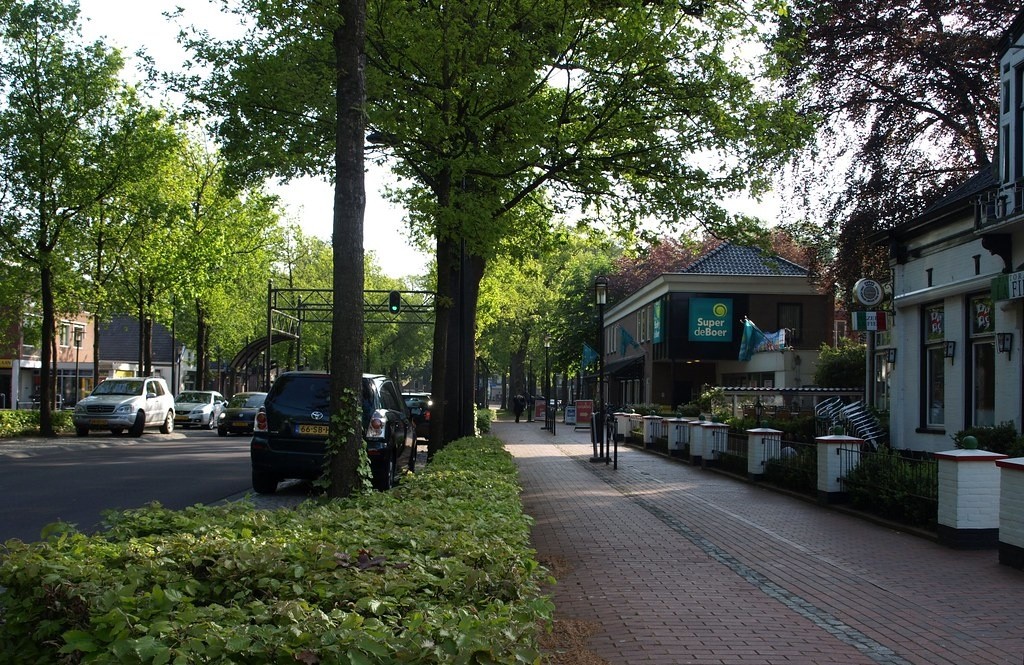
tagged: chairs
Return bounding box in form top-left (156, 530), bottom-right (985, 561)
top-left (815, 396), bottom-right (887, 454)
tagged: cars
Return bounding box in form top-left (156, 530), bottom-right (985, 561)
top-left (174, 391), bottom-right (268, 437)
top-left (72, 378), bottom-right (175, 438)
top-left (251, 371), bottom-right (433, 494)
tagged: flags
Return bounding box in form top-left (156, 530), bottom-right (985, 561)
top-left (582, 344), bottom-right (597, 368)
top-left (739, 321), bottom-right (762, 361)
top-left (619, 327), bottom-right (639, 357)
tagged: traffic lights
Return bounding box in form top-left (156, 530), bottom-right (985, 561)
top-left (389, 291), bottom-right (401, 314)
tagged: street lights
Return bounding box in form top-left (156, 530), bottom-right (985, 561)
top-left (71, 331), bottom-right (87, 403)
top-left (594, 276), bottom-right (607, 458)
top-left (544, 341), bottom-right (550, 428)
top-left (177, 345), bottom-right (184, 394)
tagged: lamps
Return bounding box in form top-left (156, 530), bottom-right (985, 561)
top-left (941, 338), bottom-right (955, 367)
top-left (754, 400), bottom-right (763, 416)
top-left (883, 346), bottom-right (896, 370)
top-left (996, 330), bottom-right (1013, 361)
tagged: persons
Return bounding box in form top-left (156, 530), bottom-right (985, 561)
top-left (513, 394), bottom-right (526, 423)
top-left (29, 385), bottom-right (40, 407)
top-left (198, 395), bottom-right (204, 402)
